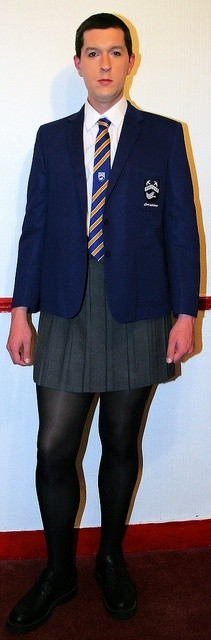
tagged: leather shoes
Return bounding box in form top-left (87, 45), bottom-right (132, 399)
top-left (95, 554), bottom-right (137, 620)
top-left (5, 568), bottom-right (78, 634)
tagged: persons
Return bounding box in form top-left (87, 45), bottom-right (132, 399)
top-left (5, 11), bottom-right (202, 633)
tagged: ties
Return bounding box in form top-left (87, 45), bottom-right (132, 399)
top-left (89, 117), bottom-right (110, 261)
